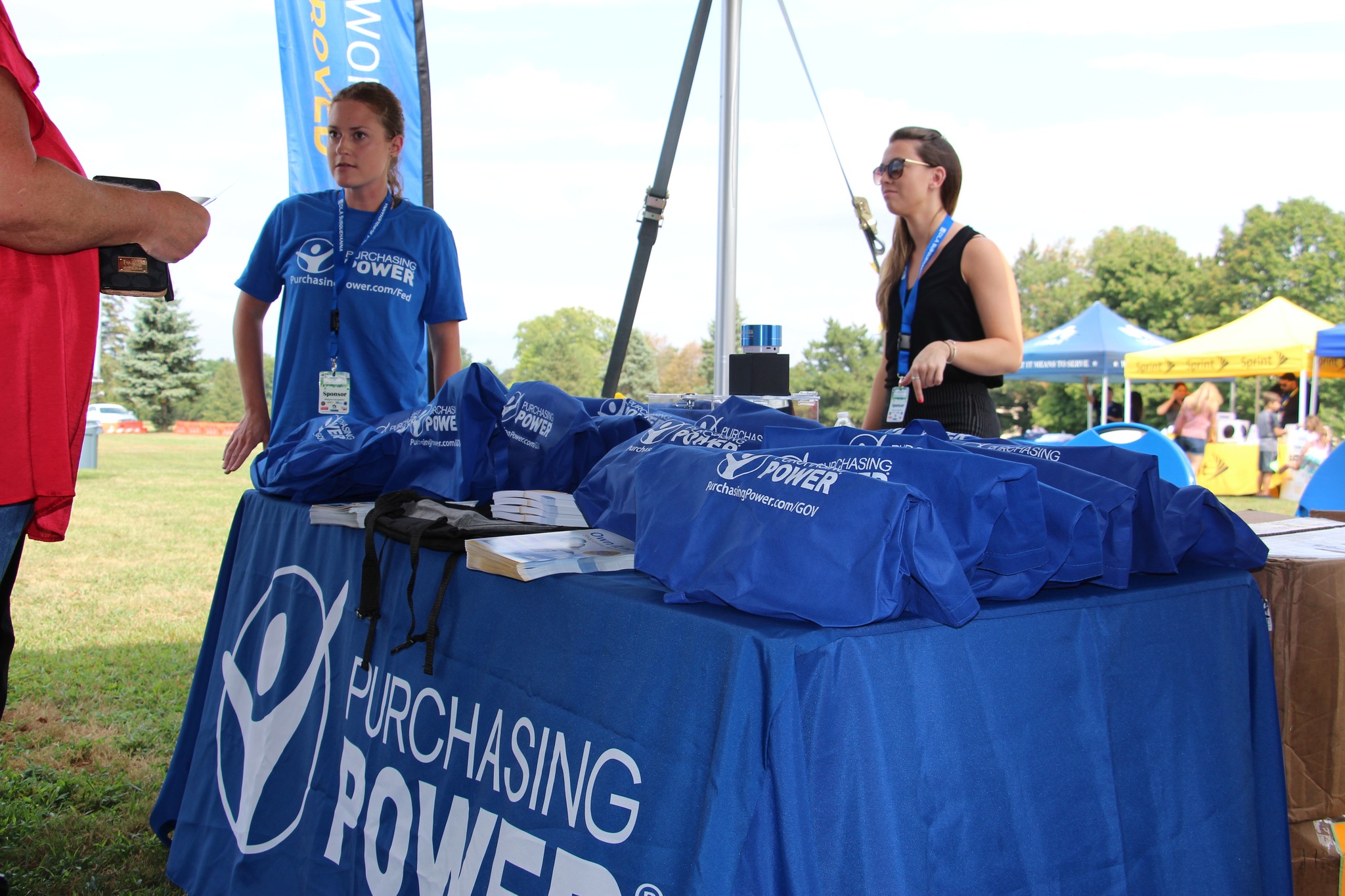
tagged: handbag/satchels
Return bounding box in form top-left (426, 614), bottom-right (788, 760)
top-left (374, 485), bottom-right (590, 555)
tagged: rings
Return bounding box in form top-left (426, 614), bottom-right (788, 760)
top-left (911, 376), bottom-right (920, 381)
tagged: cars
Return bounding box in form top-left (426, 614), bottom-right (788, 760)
top-left (85, 404), bottom-right (139, 422)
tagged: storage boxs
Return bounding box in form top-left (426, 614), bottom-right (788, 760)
top-left (1242, 513), bottom-right (1345, 826)
top-left (1285, 815), bottom-right (1340, 896)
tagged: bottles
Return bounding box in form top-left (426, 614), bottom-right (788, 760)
top-left (834, 411), bottom-right (856, 428)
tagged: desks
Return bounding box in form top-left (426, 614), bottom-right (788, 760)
top-left (141, 485), bottom-right (1301, 896)
top-left (1165, 433), bottom-right (1263, 499)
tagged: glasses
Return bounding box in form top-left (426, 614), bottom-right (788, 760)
top-left (873, 157), bottom-right (930, 185)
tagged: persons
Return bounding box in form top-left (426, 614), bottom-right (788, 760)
top-left (220, 81), bottom-right (465, 475)
top-left (1286, 413), bottom-right (1333, 499)
top-left (0, 4), bottom-right (212, 763)
top-left (1256, 390), bottom-right (1289, 496)
top-left (1155, 382), bottom-right (1192, 427)
top-left (856, 125), bottom-right (1023, 444)
top-left (1131, 392), bottom-right (1144, 424)
top-left (1081, 376), bottom-right (1123, 426)
top-left (1269, 371), bottom-right (1320, 429)
top-left (1174, 381), bottom-right (1225, 478)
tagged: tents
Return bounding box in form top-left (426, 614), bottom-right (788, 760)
top-left (1002, 299), bottom-right (1238, 437)
top-left (1124, 295), bottom-right (1344, 444)
top-left (1308, 321), bottom-right (1345, 428)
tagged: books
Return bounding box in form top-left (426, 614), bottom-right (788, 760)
top-left (307, 484), bottom-right (640, 591)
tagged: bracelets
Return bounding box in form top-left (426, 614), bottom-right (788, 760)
top-left (945, 339), bottom-right (957, 364)
top-left (941, 340), bottom-right (952, 361)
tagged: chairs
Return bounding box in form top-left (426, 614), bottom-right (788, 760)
top-left (1062, 419), bottom-right (1197, 493)
